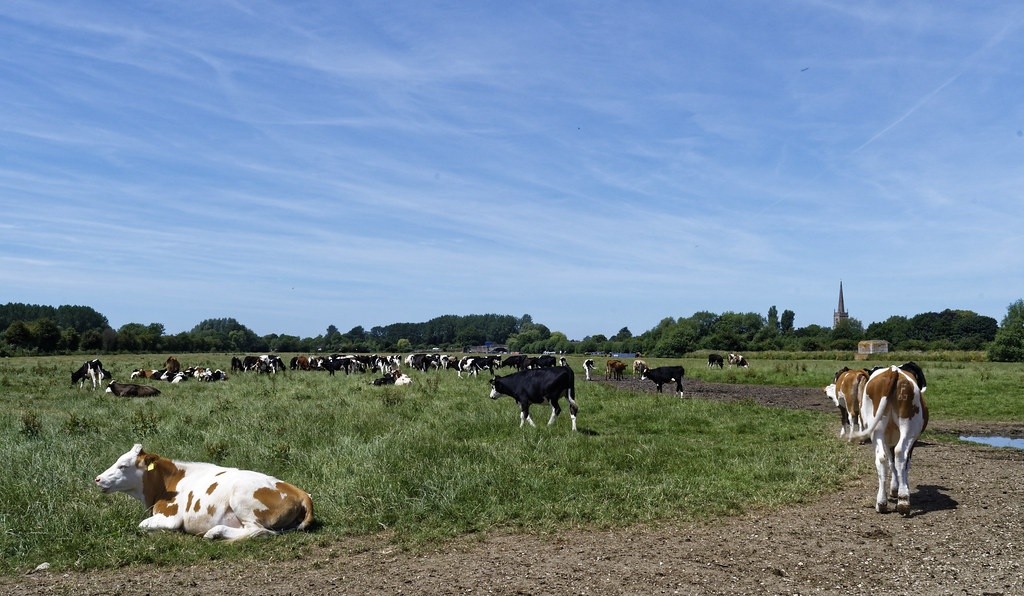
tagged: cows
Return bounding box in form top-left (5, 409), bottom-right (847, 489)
top-left (68, 353), bottom-right (752, 399)
top-left (489, 366), bottom-right (579, 432)
top-left (823, 360), bottom-right (928, 517)
top-left (94, 443), bottom-right (315, 543)
